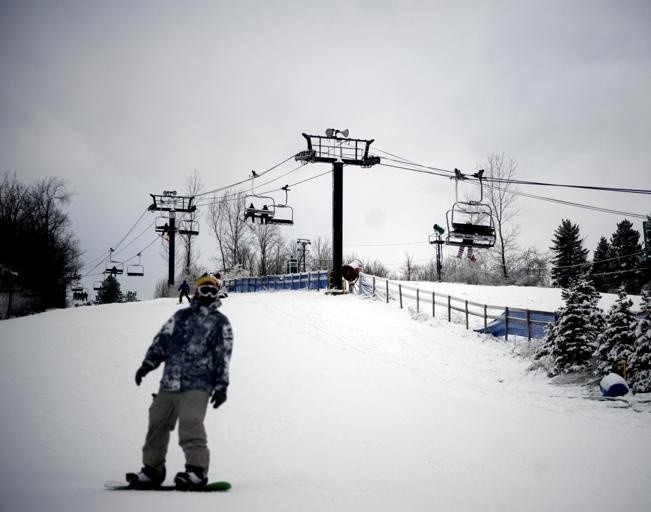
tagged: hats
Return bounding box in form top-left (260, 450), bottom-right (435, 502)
top-left (195, 273), bottom-right (223, 288)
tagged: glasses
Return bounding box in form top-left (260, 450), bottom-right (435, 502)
top-left (198, 285), bottom-right (219, 298)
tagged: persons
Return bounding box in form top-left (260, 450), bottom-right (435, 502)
top-left (245, 203), bottom-right (254, 222)
top-left (125, 273), bottom-right (233, 485)
top-left (261, 205), bottom-right (268, 224)
top-left (162, 222), bottom-right (169, 235)
top-left (456, 222), bottom-right (473, 259)
top-left (178, 280), bottom-right (191, 304)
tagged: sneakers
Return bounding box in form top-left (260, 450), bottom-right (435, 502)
top-left (176, 471), bottom-right (208, 485)
top-left (126, 465), bottom-right (165, 482)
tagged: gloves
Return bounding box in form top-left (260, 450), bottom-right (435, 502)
top-left (211, 387), bottom-right (226, 408)
top-left (136, 362), bottom-right (154, 385)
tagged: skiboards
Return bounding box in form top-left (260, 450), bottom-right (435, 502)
top-left (105, 473), bottom-right (233, 492)
